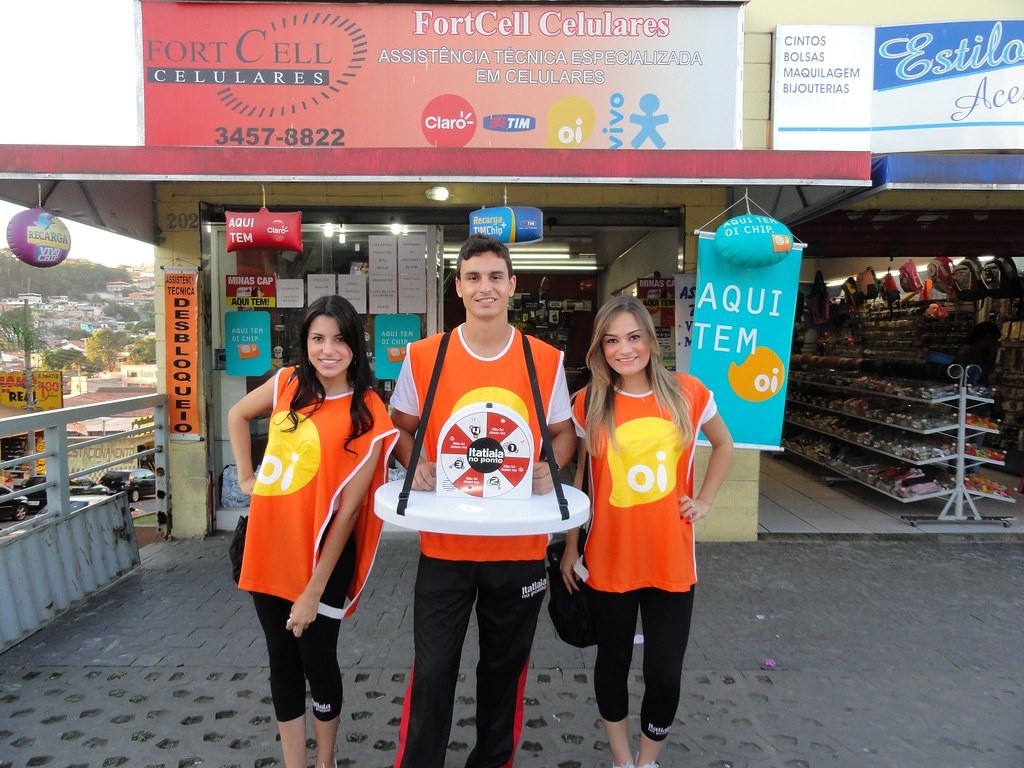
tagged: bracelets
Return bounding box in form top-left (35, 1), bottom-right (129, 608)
top-left (543, 457), bottom-right (561, 472)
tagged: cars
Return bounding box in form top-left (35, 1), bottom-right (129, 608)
top-left (0, 485), bottom-right (30, 521)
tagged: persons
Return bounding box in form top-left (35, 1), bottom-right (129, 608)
top-left (387, 235), bottom-right (578, 768)
top-left (227, 296), bottom-right (400, 768)
top-left (553, 295), bottom-right (732, 768)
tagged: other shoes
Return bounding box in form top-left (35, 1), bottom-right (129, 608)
top-left (635, 751), bottom-right (660, 768)
top-left (613, 760), bottom-right (635, 768)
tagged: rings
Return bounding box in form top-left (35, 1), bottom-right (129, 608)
top-left (689, 502), bottom-right (695, 508)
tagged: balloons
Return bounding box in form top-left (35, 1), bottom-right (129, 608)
top-left (8, 207), bottom-right (71, 267)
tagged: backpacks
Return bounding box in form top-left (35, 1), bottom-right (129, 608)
top-left (806, 294), bottom-right (832, 325)
top-left (929, 256), bottom-right (955, 294)
top-left (899, 259), bottom-right (923, 292)
top-left (877, 274), bottom-right (900, 305)
top-left (857, 266), bottom-right (879, 303)
top-left (952, 256), bottom-right (984, 311)
top-left (842, 277), bottom-right (864, 306)
top-left (981, 255), bottom-right (1018, 300)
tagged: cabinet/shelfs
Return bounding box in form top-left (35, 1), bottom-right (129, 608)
top-left (773, 364), bottom-right (1017, 528)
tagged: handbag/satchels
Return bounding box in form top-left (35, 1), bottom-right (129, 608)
top-left (230, 516), bottom-right (248, 583)
top-left (546, 526), bottom-right (596, 648)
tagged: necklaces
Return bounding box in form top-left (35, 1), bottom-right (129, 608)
top-left (617, 380), bottom-right (647, 403)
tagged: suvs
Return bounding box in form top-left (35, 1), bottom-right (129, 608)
top-left (99, 468), bottom-right (156, 502)
top-left (19, 472), bottom-right (114, 512)
